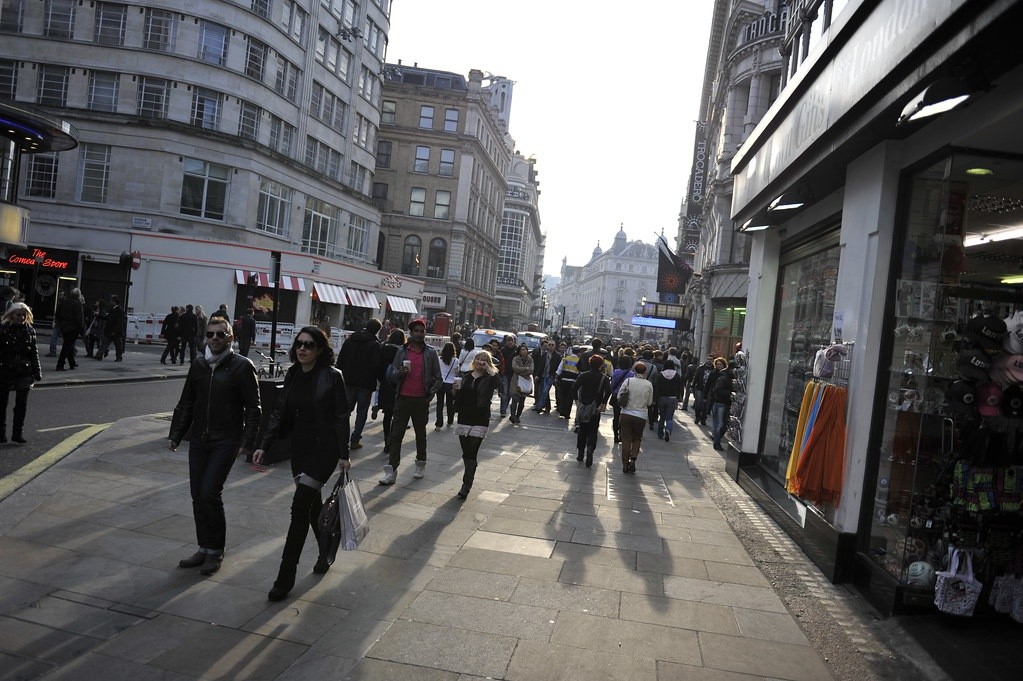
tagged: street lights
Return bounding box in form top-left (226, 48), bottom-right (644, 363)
top-left (539, 286), bottom-right (548, 330)
top-left (558, 312), bottom-right (561, 328)
top-left (640, 295), bottom-right (646, 339)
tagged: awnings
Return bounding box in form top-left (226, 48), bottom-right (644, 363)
top-left (387, 295), bottom-right (418, 313)
top-left (346, 289), bottom-right (380, 309)
top-left (313, 282), bottom-right (348, 305)
top-left (234, 270), bottom-right (306, 291)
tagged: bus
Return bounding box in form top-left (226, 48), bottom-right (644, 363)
top-left (622, 324), bottom-right (640, 339)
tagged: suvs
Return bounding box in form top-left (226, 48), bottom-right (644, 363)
top-left (461, 328), bottom-right (549, 355)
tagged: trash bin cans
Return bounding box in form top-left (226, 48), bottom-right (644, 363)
top-left (245, 377), bottom-right (289, 465)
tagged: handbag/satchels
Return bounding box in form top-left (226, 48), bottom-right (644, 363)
top-left (575, 402), bottom-right (596, 423)
top-left (232, 316), bottom-right (244, 337)
top-left (516, 376), bottom-right (532, 395)
top-left (84, 328), bottom-right (90, 336)
top-left (618, 378), bottom-right (631, 406)
top-left (936, 550), bottom-right (983, 616)
top-left (318, 468), bottom-right (370, 550)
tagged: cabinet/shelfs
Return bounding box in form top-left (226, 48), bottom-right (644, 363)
top-left (865, 257), bottom-right (1023, 615)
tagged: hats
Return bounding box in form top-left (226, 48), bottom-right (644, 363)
top-left (408, 320), bottom-right (425, 329)
top-left (947, 311), bottom-right (1023, 417)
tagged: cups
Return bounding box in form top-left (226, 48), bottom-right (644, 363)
top-left (403, 360), bottom-right (411, 372)
top-left (454, 376), bottom-right (462, 389)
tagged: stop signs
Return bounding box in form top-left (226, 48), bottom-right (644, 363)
top-left (131, 249), bottom-right (142, 271)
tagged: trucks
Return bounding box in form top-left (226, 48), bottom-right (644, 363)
top-left (558, 325), bottom-right (585, 339)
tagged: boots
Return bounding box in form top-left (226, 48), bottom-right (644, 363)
top-left (310, 494), bottom-right (336, 574)
top-left (12, 406), bottom-right (27, 442)
top-left (414, 460), bottom-right (427, 477)
top-left (268, 483), bottom-right (318, 598)
top-left (379, 464), bottom-right (398, 484)
top-left (458, 460), bottom-right (477, 497)
top-left (0, 404), bottom-right (8, 443)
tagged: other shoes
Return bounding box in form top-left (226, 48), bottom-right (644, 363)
top-left (44, 348), bottom-right (197, 371)
top-left (350, 442), bottom-right (362, 448)
top-left (201, 550), bottom-right (223, 573)
top-left (435, 405), bottom-right (725, 471)
top-left (179, 550), bottom-right (207, 568)
top-left (371, 405), bottom-right (379, 420)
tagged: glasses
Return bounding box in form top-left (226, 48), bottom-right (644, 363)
top-left (548, 344), bottom-right (555, 347)
top-left (206, 331), bottom-right (230, 339)
top-left (294, 340), bottom-right (321, 351)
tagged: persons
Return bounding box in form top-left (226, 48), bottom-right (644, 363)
top-left (712, 359), bottom-right (735, 451)
top-left (483, 331), bottom-right (727, 445)
top-left (0, 303), bottom-right (42, 443)
top-left (571, 355), bottom-right (611, 466)
top-left (253, 326), bottom-right (351, 601)
top-left (378, 319), bottom-right (443, 485)
top-left (210, 304), bottom-right (230, 323)
top-left (336, 318), bottom-right (407, 453)
top-left (237, 308), bottom-right (257, 357)
top-left (435, 332), bottom-right (476, 431)
top-left (318, 315), bottom-right (331, 338)
top-left (159, 305), bottom-right (208, 365)
top-left (168, 316), bottom-right (262, 575)
top-left (452, 350), bottom-right (500, 497)
top-left (0, 285), bottom-right (26, 316)
top-left (617, 362), bottom-right (653, 473)
top-left (45, 288), bottom-right (127, 371)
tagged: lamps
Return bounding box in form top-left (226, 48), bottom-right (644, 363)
top-left (897, 78), bottom-right (978, 125)
top-left (740, 217), bottom-right (769, 232)
top-left (767, 192), bottom-right (804, 211)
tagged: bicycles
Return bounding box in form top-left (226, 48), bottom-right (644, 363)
top-left (255, 349), bottom-right (288, 379)
